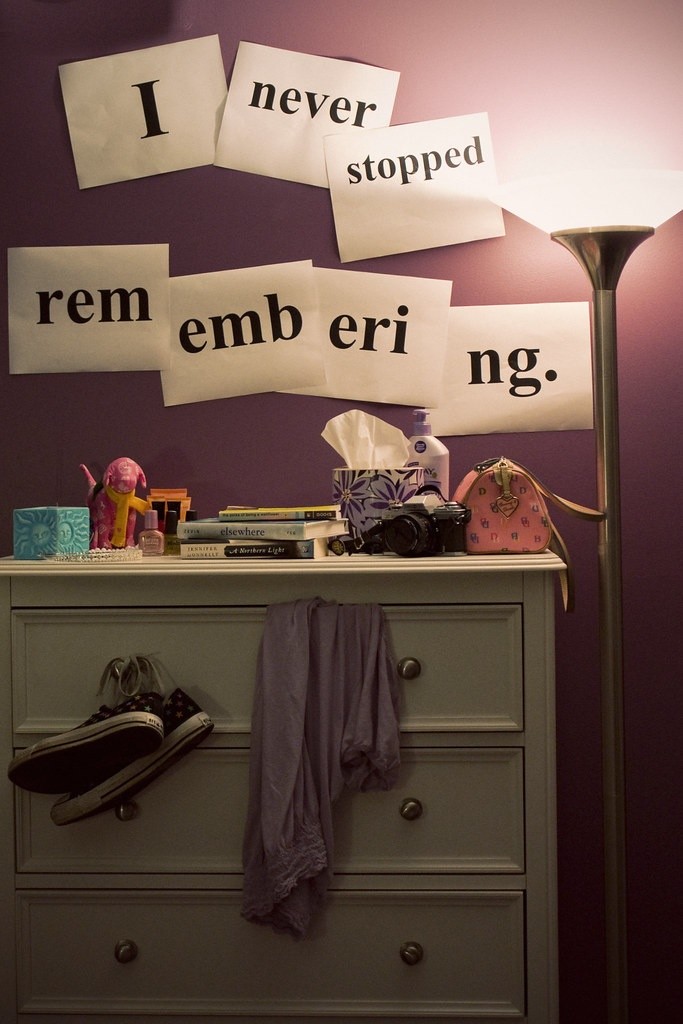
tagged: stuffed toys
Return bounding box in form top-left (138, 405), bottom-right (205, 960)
top-left (79, 457), bottom-right (148, 550)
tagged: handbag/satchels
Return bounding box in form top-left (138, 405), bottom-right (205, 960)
top-left (452, 454), bottom-right (606, 616)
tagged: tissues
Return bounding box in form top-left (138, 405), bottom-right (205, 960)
top-left (319, 409), bottom-right (425, 555)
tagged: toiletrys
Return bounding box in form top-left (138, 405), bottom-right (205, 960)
top-left (407, 410), bottom-right (450, 502)
top-left (137, 488), bottom-right (198, 557)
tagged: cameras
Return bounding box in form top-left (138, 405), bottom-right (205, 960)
top-left (377, 494), bottom-right (472, 557)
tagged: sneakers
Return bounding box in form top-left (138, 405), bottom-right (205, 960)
top-left (49, 684), bottom-right (215, 827)
top-left (6, 691), bottom-right (164, 796)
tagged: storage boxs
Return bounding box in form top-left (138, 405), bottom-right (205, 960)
top-left (13, 507), bottom-right (90, 560)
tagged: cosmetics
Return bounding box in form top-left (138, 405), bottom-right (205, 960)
top-left (137, 488), bottom-right (198, 557)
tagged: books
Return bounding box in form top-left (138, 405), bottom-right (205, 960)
top-left (177, 503), bottom-right (350, 559)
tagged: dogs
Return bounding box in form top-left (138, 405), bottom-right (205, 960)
top-left (77, 456), bottom-right (147, 550)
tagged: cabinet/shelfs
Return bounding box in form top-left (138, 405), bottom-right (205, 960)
top-left (1, 548), bottom-right (566, 1024)
